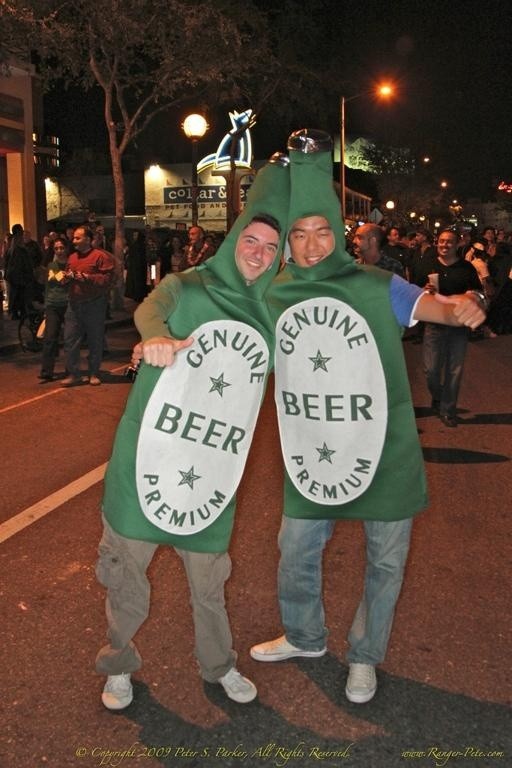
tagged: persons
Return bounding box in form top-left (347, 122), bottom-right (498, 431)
top-left (97, 162), bottom-right (289, 711)
top-left (129, 152), bottom-right (487, 704)
top-left (0, 212), bottom-right (511, 428)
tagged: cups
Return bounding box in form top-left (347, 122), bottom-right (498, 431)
top-left (427, 273), bottom-right (440, 294)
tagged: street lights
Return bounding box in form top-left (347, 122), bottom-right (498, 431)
top-left (339, 73), bottom-right (403, 223)
top-left (181, 110), bottom-right (212, 231)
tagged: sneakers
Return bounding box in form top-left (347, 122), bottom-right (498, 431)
top-left (345, 662), bottom-right (377, 704)
top-left (61, 374), bottom-right (81, 386)
top-left (89, 374), bottom-right (100, 385)
top-left (220, 667), bottom-right (257, 703)
top-left (249, 634), bottom-right (327, 662)
top-left (102, 672), bottom-right (134, 711)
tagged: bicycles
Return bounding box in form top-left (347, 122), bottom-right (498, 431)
top-left (18, 304), bottom-right (68, 353)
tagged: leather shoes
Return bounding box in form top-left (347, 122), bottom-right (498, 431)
top-left (440, 416), bottom-right (458, 427)
